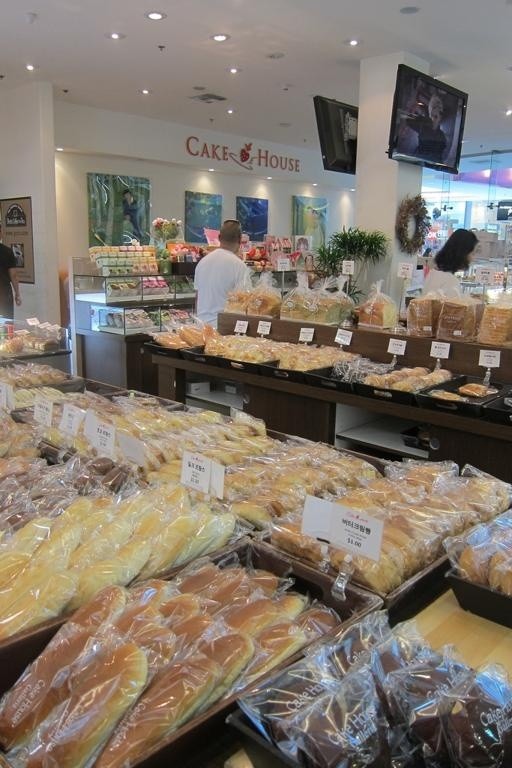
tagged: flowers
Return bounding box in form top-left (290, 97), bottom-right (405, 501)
top-left (395, 193), bottom-right (434, 255)
top-left (147, 216), bottom-right (184, 243)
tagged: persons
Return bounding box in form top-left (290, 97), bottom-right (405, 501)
top-left (0, 244), bottom-right (22, 322)
top-left (423, 248), bottom-right (432, 277)
top-left (194, 218), bottom-right (250, 329)
top-left (406, 96), bottom-right (452, 165)
top-left (420, 228), bottom-right (478, 305)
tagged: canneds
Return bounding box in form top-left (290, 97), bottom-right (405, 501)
top-left (176, 256), bottom-right (184, 262)
top-left (184, 255), bottom-right (192, 262)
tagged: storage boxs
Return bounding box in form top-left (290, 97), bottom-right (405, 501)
top-left (469, 230), bottom-right (505, 286)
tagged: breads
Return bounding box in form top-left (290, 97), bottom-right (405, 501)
top-left (1, 291), bottom-right (512, 768)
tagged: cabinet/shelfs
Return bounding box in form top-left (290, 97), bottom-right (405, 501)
top-left (68, 254), bottom-right (199, 406)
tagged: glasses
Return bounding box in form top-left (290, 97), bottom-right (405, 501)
top-left (223, 219), bottom-right (240, 224)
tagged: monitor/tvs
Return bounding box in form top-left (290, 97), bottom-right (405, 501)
top-left (312, 95), bottom-right (359, 174)
top-left (388, 63), bottom-right (469, 174)
